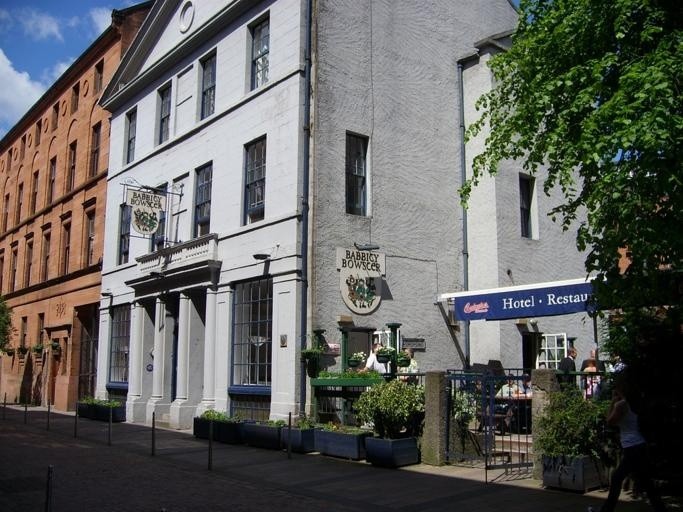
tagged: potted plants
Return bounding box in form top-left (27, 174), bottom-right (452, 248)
top-left (301, 349), bottom-right (321, 377)
top-left (194, 410), bottom-right (374, 460)
top-left (348, 344), bottom-right (411, 367)
top-left (532, 380), bottom-right (621, 492)
top-left (351, 379), bottom-right (424, 467)
top-left (3, 344), bottom-right (44, 356)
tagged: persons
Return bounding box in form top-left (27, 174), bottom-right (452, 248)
top-left (399, 348), bottom-right (418, 384)
top-left (496, 373), bottom-right (522, 420)
top-left (579, 349), bottom-right (604, 391)
top-left (519, 373), bottom-right (533, 396)
top-left (557, 346), bottom-right (578, 392)
top-left (586, 376), bottom-right (668, 512)
top-left (362, 340), bottom-right (391, 373)
